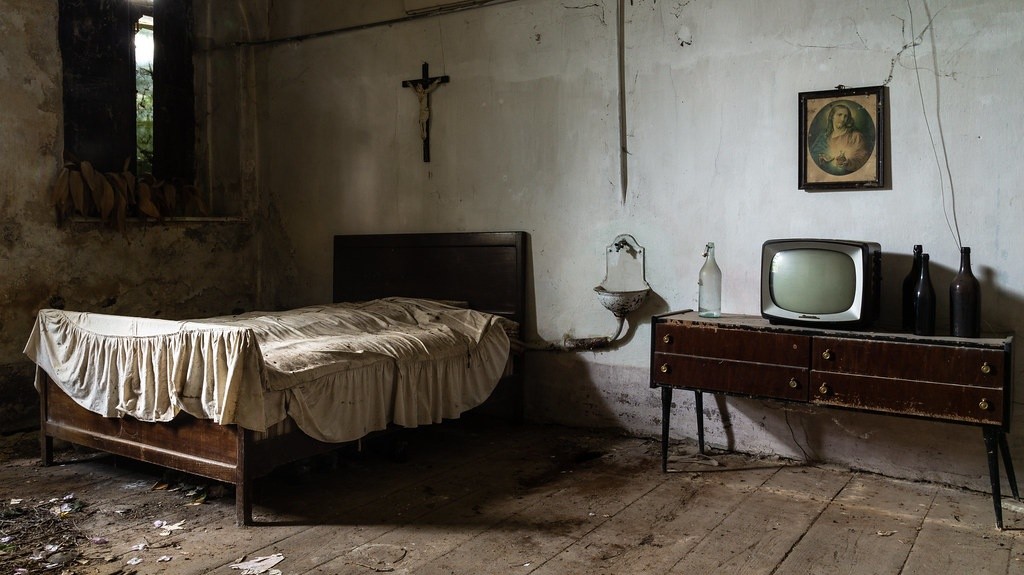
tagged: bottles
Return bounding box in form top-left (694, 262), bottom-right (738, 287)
top-left (950, 247), bottom-right (981, 339)
top-left (698, 242), bottom-right (722, 317)
top-left (903, 245), bottom-right (936, 336)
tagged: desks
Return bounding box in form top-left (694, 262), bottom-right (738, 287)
top-left (648, 309), bottom-right (1024, 532)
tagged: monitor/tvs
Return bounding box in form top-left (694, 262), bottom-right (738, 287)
top-left (761, 239), bottom-right (883, 331)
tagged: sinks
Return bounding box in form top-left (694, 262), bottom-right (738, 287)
top-left (593, 277), bottom-right (650, 312)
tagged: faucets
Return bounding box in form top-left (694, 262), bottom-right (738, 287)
top-left (614, 240), bottom-right (626, 253)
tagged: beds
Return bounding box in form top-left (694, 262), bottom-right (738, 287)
top-left (26, 229), bottom-right (528, 524)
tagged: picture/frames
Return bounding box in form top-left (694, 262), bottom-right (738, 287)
top-left (799, 85), bottom-right (886, 191)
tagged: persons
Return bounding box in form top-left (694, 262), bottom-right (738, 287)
top-left (407, 78), bottom-right (441, 139)
